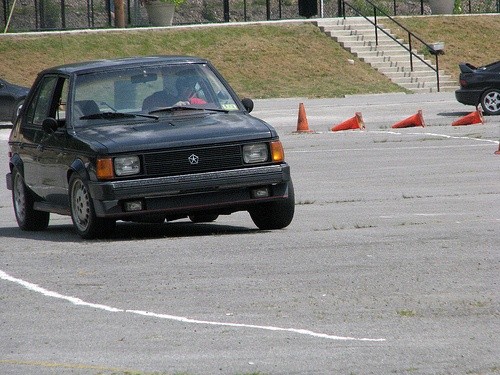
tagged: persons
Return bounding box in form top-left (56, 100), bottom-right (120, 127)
top-left (148, 67), bottom-right (206, 112)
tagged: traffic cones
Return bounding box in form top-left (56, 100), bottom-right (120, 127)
top-left (450, 106), bottom-right (484, 126)
top-left (495, 139), bottom-right (500, 155)
top-left (292, 102), bottom-right (315, 132)
top-left (391, 109), bottom-right (426, 129)
top-left (331, 111), bottom-right (366, 132)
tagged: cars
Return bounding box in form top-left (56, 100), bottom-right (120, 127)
top-left (454, 59), bottom-right (500, 115)
top-left (0, 76), bottom-right (32, 127)
top-left (6, 54), bottom-right (296, 240)
